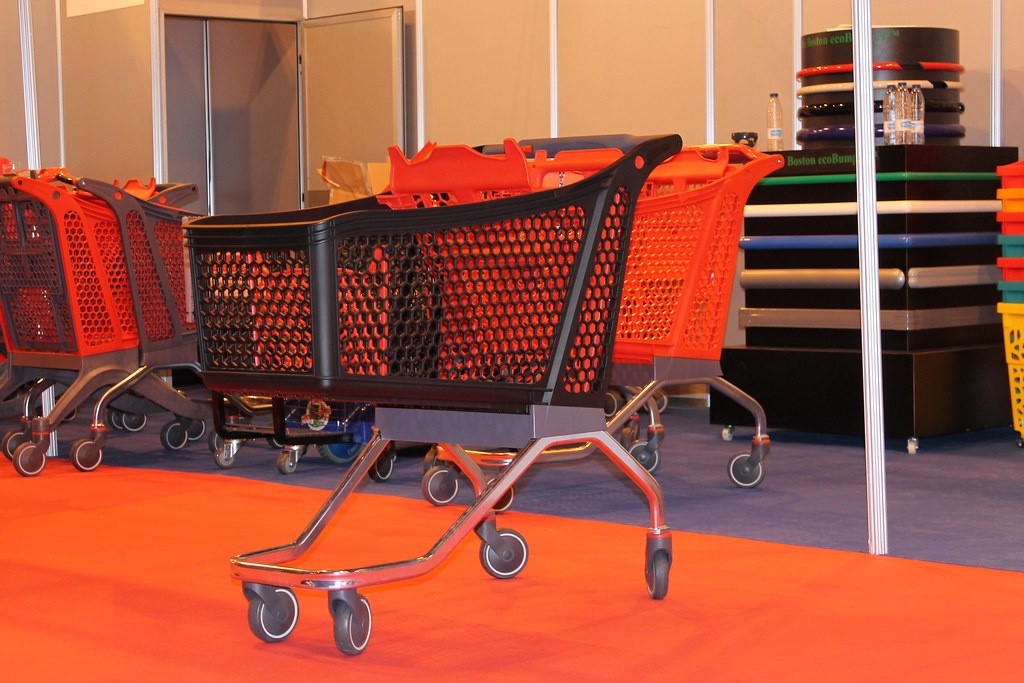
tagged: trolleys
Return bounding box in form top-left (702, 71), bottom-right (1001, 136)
top-left (421, 139), bottom-right (773, 515)
top-left (0, 156), bottom-right (429, 485)
top-left (195, 133), bottom-right (673, 656)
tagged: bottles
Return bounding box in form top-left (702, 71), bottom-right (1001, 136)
top-left (767, 92), bottom-right (783, 151)
top-left (911, 85), bottom-right (925, 144)
top-left (883, 85), bottom-right (900, 145)
top-left (897, 82), bottom-right (912, 144)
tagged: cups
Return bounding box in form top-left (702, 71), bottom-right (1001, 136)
top-left (732, 132), bottom-right (758, 148)
